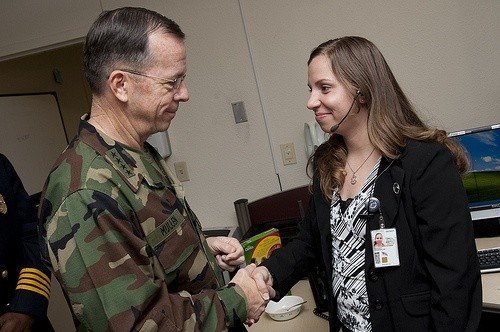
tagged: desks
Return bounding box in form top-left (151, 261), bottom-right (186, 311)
top-left (475, 236), bottom-right (500, 314)
top-left (243, 280), bottom-right (342, 332)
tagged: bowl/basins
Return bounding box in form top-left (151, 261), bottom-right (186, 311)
top-left (264, 295), bottom-right (304, 320)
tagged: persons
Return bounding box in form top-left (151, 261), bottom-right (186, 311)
top-left (0, 153), bottom-right (55, 332)
top-left (374, 233), bottom-right (384, 248)
top-left (244, 36), bottom-right (482, 332)
top-left (38, 7), bottom-right (276, 332)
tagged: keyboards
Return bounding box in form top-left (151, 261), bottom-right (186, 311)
top-left (477, 247), bottom-right (500, 273)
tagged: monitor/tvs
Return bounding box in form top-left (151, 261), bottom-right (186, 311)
top-left (444, 123), bottom-right (500, 211)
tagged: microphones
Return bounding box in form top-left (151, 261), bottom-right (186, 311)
top-left (330, 86), bottom-right (361, 133)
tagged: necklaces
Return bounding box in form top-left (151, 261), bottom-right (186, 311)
top-left (346, 147), bottom-right (375, 185)
